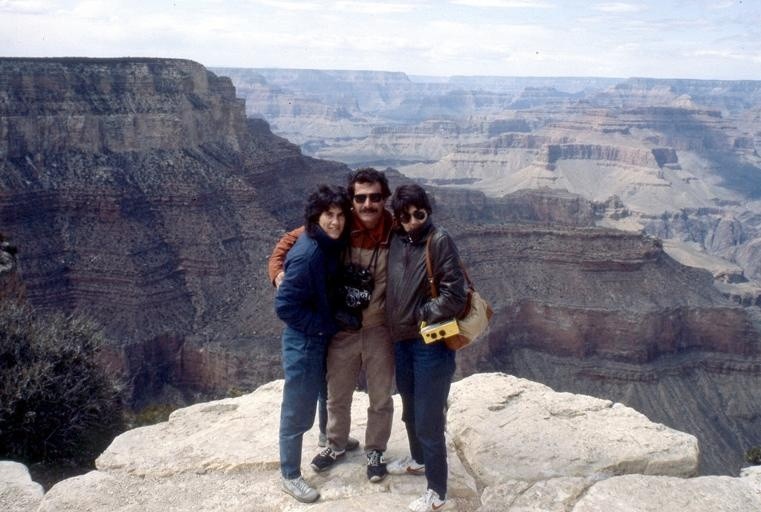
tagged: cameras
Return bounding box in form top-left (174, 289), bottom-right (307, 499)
top-left (339, 286), bottom-right (371, 308)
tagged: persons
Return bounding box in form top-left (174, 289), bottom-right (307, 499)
top-left (383, 182), bottom-right (470, 512)
top-left (265, 163), bottom-right (404, 485)
top-left (271, 182), bottom-right (360, 506)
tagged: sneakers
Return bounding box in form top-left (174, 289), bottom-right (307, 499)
top-left (367, 450), bottom-right (386, 482)
top-left (318, 432), bottom-right (359, 450)
top-left (310, 448), bottom-right (346, 472)
top-left (409, 489), bottom-right (447, 512)
top-left (281, 476), bottom-right (320, 503)
top-left (387, 458), bottom-right (426, 475)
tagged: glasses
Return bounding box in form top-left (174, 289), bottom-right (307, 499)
top-left (354, 193), bottom-right (382, 202)
top-left (400, 211), bottom-right (425, 223)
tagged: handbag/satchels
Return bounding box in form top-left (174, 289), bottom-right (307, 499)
top-left (445, 290), bottom-right (492, 350)
